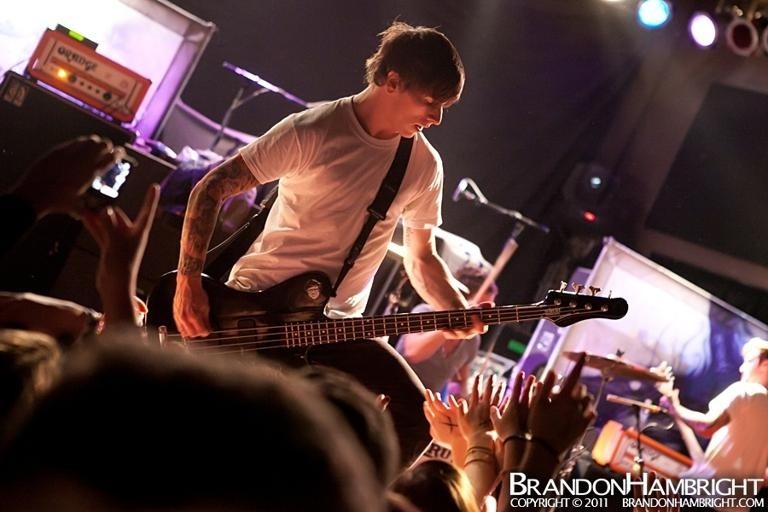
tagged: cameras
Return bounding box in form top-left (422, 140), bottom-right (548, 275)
top-left (90, 162), bottom-right (132, 200)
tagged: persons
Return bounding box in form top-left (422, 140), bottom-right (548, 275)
top-left (1, 125), bottom-right (768, 511)
top-left (169, 19), bottom-right (497, 354)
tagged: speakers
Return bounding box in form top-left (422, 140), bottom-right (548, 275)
top-left (1, 69), bottom-right (137, 207)
top-left (113, 142), bottom-right (178, 221)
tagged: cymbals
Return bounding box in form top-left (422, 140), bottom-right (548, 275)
top-left (561, 350), bottom-right (670, 382)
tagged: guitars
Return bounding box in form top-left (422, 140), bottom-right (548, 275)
top-left (144, 269), bottom-right (629, 368)
top-left (648, 361), bottom-right (704, 462)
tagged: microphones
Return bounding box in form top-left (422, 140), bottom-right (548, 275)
top-left (451, 177), bottom-right (521, 219)
top-left (605, 393), bottom-right (668, 419)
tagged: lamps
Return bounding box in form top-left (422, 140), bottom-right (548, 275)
top-left (689, 0), bottom-right (768, 58)
